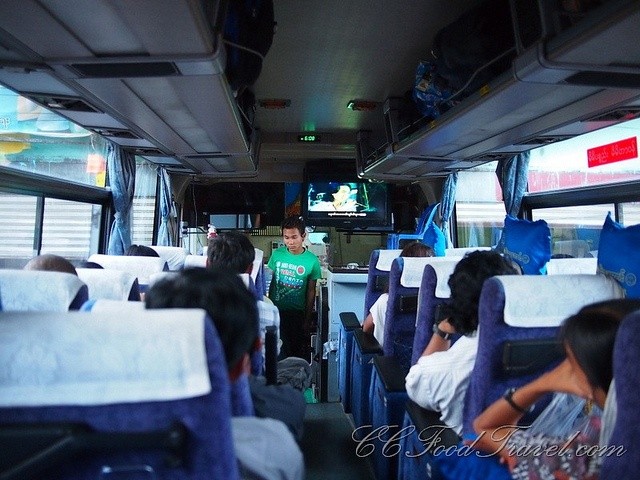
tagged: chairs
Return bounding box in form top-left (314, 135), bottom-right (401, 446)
top-left (0, 308), bottom-right (237, 479)
top-left (348, 256), bottom-right (464, 479)
top-left (338, 250), bottom-right (405, 415)
top-left (368, 260), bottom-right (464, 480)
top-left (386, 202), bottom-right (440, 250)
top-left (397, 275), bottom-right (625, 480)
top-left (596, 311), bottom-right (639, 478)
top-left (0, 246), bottom-right (262, 309)
top-left (445, 246), bottom-right (492, 256)
top-left (545, 256), bottom-right (598, 276)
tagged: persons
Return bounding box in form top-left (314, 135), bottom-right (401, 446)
top-left (123, 244), bottom-right (161, 257)
top-left (362, 242), bottom-right (436, 347)
top-left (404, 250), bottom-right (519, 439)
top-left (205, 233), bottom-right (306, 443)
top-left (145, 268), bottom-right (308, 479)
top-left (313, 184), bottom-right (355, 214)
top-left (462, 296), bottom-right (639, 479)
top-left (22, 252), bottom-right (79, 278)
top-left (257, 298), bottom-right (312, 393)
top-left (267, 218), bottom-right (323, 362)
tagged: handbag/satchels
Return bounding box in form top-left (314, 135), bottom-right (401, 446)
top-left (223, 0), bottom-right (273, 88)
top-left (232, 89), bottom-right (255, 135)
top-left (412, 61), bottom-right (460, 119)
top-left (427, 2), bottom-right (542, 97)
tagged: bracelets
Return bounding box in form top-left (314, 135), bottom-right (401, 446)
top-left (434, 325), bottom-right (452, 339)
top-left (504, 387), bottom-right (531, 416)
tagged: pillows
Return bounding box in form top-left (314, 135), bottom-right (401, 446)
top-left (503, 215), bottom-right (552, 276)
top-left (422, 222), bottom-right (445, 256)
top-left (596, 210), bottom-right (639, 299)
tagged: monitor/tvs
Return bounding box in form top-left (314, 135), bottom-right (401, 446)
top-left (335, 212), bottom-right (396, 236)
top-left (182, 184), bottom-right (253, 229)
top-left (215, 182), bottom-right (285, 230)
top-left (301, 183), bottom-right (388, 227)
